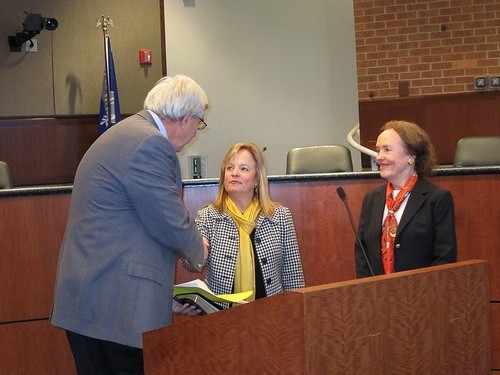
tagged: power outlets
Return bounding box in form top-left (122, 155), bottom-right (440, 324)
top-left (25, 38), bottom-right (38, 53)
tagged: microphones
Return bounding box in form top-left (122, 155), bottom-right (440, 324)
top-left (336, 186), bottom-right (375, 276)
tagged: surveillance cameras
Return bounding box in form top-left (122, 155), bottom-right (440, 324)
top-left (44, 17), bottom-right (58, 31)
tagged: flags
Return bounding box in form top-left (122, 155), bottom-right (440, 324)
top-left (99, 38), bottom-right (121, 134)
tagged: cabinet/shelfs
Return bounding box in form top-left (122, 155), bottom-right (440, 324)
top-left (0, 0), bottom-right (162, 118)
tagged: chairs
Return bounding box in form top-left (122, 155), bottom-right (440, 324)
top-left (453, 136), bottom-right (500, 168)
top-left (286, 144), bottom-right (353, 174)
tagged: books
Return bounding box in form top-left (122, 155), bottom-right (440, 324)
top-left (174, 292), bottom-right (224, 315)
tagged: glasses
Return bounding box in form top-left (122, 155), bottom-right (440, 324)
top-left (197, 117), bottom-right (207, 130)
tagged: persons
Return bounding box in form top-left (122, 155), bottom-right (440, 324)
top-left (179, 142), bottom-right (305, 310)
top-left (49, 75), bottom-right (208, 375)
top-left (354, 121), bottom-right (458, 279)
